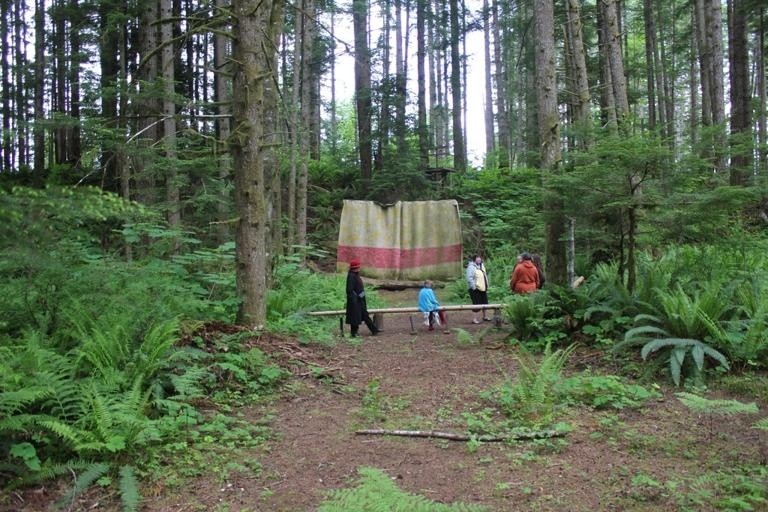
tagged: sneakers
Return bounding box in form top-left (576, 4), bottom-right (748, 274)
top-left (483, 317), bottom-right (492, 322)
top-left (352, 332), bottom-right (360, 337)
top-left (429, 326), bottom-right (435, 330)
top-left (472, 318), bottom-right (480, 324)
top-left (443, 328), bottom-right (449, 332)
top-left (373, 329), bottom-right (383, 334)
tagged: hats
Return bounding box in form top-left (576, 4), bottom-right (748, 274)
top-left (521, 252), bottom-right (531, 260)
top-left (349, 260), bottom-right (360, 269)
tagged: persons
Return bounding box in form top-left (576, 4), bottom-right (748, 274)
top-left (530, 253), bottom-right (547, 290)
top-left (343, 260), bottom-right (384, 337)
top-left (509, 256), bottom-right (520, 280)
top-left (507, 251), bottom-right (539, 298)
top-left (416, 278), bottom-right (449, 331)
top-left (464, 253), bottom-right (492, 324)
